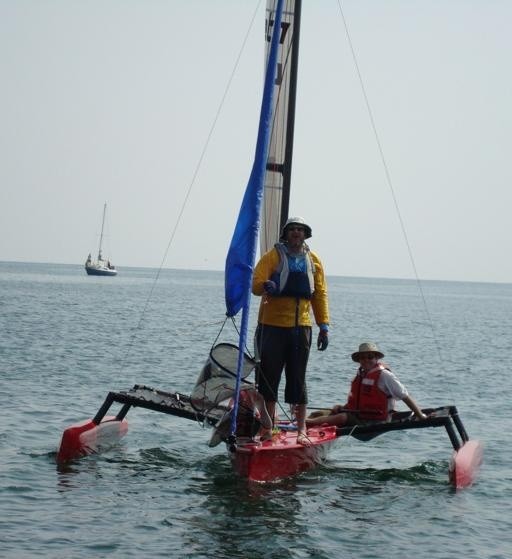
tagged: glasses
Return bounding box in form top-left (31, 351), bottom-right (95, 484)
top-left (288, 227), bottom-right (305, 231)
top-left (359, 354), bottom-right (374, 359)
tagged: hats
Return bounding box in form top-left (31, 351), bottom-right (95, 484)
top-left (279, 217), bottom-right (312, 240)
top-left (352, 343), bottom-right (384, 362)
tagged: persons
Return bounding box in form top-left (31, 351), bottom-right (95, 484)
top-left (252, 217), bottom-right (330, 445)
top-left (306, 342), bottom-right (437, 442)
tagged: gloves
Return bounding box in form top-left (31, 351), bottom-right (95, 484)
top-left (317, 324), bottom-right (328, 351)
top-left (263, 280), bottom-right (276, 295)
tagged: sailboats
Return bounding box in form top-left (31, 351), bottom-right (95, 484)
top-left (86, 202), bottom-right (119, 276)
top-left (56, 0), bottom-right (480, 490)
